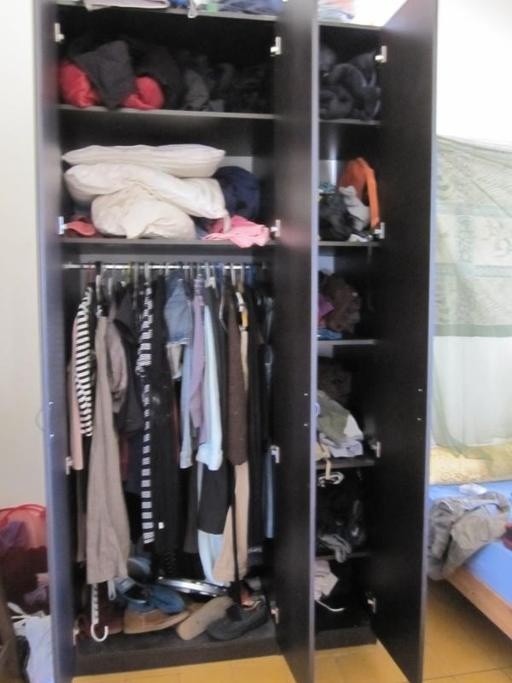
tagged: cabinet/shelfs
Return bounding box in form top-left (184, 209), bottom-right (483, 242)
top-left (30, 0), bottom-right (439, 683)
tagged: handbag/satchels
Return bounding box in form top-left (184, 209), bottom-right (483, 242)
top-left (342, 156), bottom-right (380, 230)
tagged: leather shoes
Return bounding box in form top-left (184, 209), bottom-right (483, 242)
top-left (125, 608), bottom-right (190, 633)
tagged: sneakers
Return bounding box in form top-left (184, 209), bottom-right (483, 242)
top-left (123, 581), bottom-right (184, 614)
top-left (207, 602), bottom-right (271, 639)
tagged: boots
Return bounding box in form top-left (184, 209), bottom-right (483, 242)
top-left (171, 596), bottom-right (234, 641)
top-left (127, 546), bottom-right (184, 581)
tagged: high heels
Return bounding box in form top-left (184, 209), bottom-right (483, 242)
top-left (237, 580), bottom-right (255, 606)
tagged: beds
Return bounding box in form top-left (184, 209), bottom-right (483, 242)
top-left (427, 438), bottom-right (512, 641)
